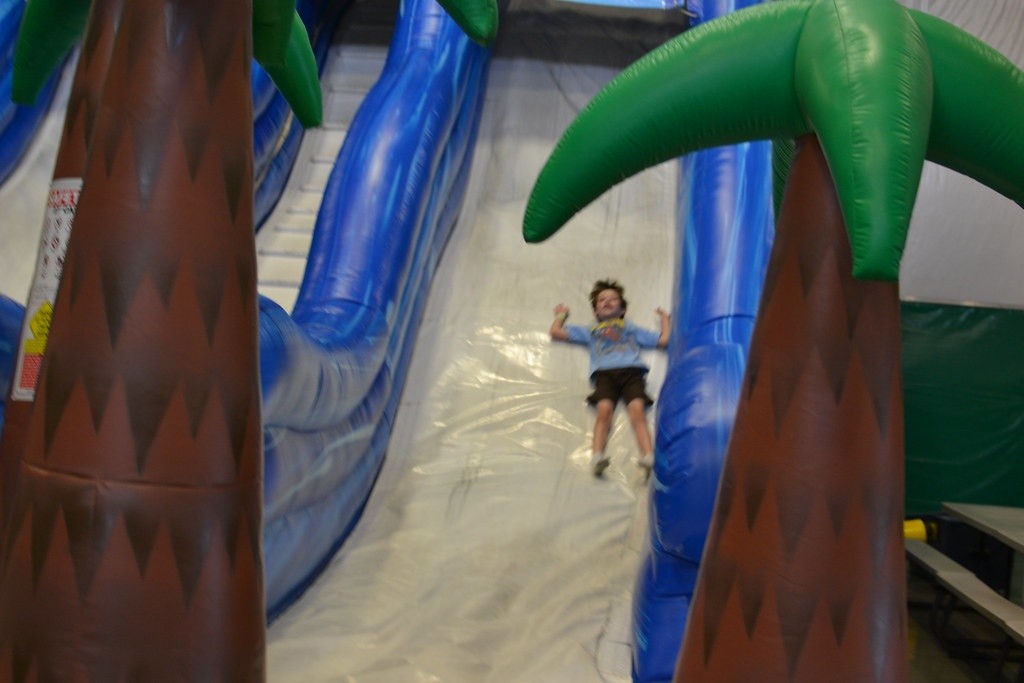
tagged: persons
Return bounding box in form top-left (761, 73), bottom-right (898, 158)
top-left (549, 280), bottom-right (671, 475)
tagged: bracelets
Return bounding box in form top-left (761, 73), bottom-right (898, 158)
top-left (556, 313), bottom-right (567, 320)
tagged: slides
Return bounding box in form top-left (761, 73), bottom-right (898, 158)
top-left (0, 0), bottom-right (777, 683)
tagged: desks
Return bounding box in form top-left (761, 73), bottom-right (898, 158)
top-left (933, 502), bottom-right (1024, 675)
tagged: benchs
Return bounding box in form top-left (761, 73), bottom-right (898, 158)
top-left (906, 538), bottom-right (1024, 682)
